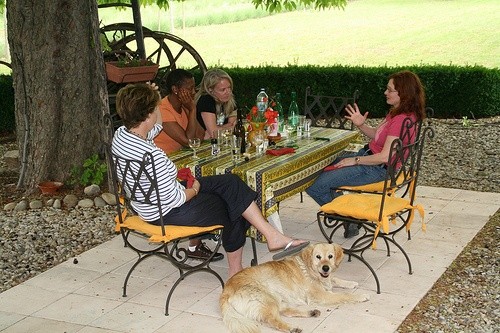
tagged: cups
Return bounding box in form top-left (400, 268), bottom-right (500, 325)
top-left (228, 136), bottom-right (241, 159)
top-left (210, 129), bottom-right (230, 156)
top-left (295, 115), bottom-right (312, 134)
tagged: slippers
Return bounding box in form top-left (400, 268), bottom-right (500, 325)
top-left (273, 240), bottom-right (310, 260)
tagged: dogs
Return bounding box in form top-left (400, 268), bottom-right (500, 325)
top-left (218, 241), bottom-right (372, 333)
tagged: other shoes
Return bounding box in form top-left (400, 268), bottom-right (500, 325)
top-left (343, 221), bottom-right (359, 238)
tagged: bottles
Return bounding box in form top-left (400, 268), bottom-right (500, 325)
top-left (275, 93), bottom-right (284, 132)
top-left (233, 109), bottom-right (245, 153)
top-left (288, 91), bottom-right (299, 127)
top-left (257, 88), bottom-right (268, 117)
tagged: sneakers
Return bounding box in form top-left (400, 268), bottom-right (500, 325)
top-left (187, 242), bottom-right (224, 262)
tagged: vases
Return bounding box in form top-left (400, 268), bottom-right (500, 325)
top-left (248, 122), bottom-right (266, 145)
top-left (37, 181), bottom-right (64, 193)
top-left (105, 60), bottom-right (160, 84)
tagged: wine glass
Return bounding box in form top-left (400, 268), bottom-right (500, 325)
top-left (284, 125), bottom-right (295, 145)
top-left (188, 137), bottom-right (200, 160)
top-left (252, 135), bottom-right (264, 158)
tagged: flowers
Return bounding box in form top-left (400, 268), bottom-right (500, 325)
top-left (246, 98), bottom-right (279, 126)
top-left (114, 51), bottom-right (155, 67)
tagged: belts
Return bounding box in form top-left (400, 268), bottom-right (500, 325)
top-left (364, 144), bottom-right (387, 169)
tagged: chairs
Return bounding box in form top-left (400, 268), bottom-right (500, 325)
top-left (104, 87), bottom-right (434, 317)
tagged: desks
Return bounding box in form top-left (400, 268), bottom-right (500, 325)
top-left (166, 120), bottom-right (359, 268)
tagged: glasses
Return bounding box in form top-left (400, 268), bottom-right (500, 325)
top-left (387, 89), bottom-right (398, 93)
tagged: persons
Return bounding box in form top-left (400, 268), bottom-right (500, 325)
top-left (151, 69), bottom-right (224, 262)
top-left (112, 81), bottom-right (310, 278)
top-left (305, 70), bottom-right (426, 238)
top-left (194, 69), bottom-right (238, 140)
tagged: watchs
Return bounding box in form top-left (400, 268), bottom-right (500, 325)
top-left (355, 156), bottom-right (361, 165)
top-left (192, 187), bottom-right (198, 196)
